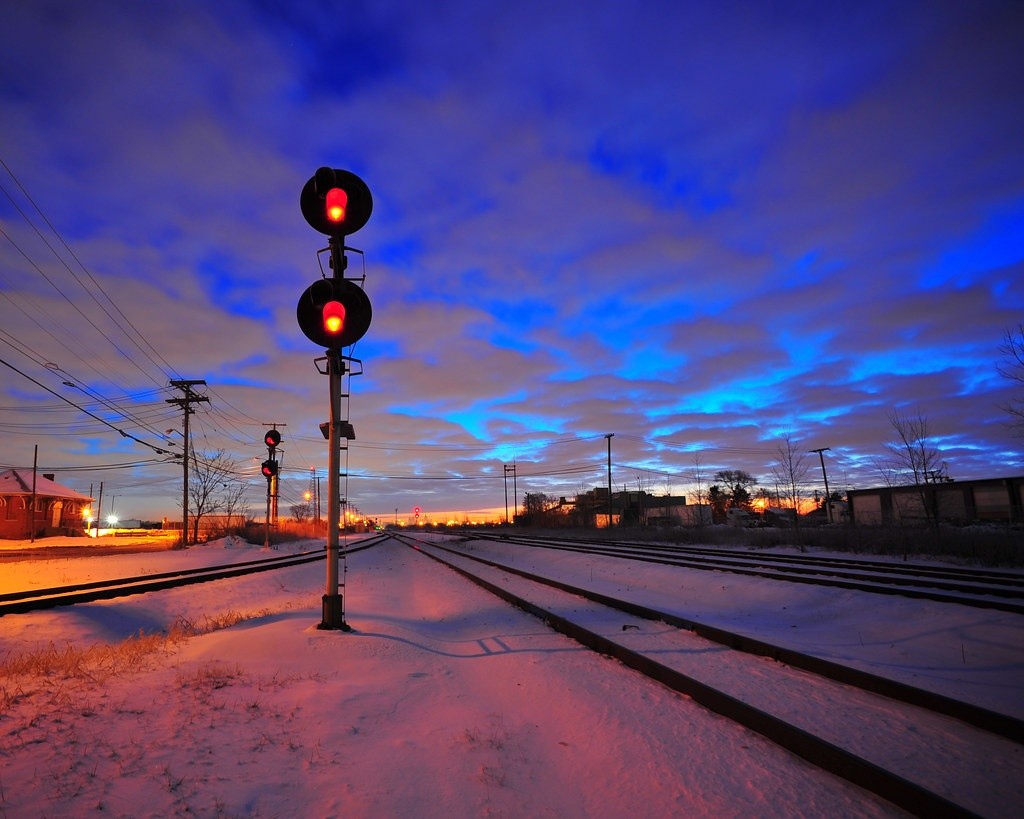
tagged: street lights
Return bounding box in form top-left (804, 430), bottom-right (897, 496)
top-left (309, 465), bottom-right (316, 520)
top-left (105, 494), bottom-right (122, 515)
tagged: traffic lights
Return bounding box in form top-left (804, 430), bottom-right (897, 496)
top-left (265, 430), bottom-right (277, 446)
top-left (309, 279), bottom-right (346, 345)
top-left (261, 460), bottom-right (273, 477)
top-left (313, 165), bottom-right (349, 231)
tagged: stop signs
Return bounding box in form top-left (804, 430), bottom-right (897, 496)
top-left (415, 514), bottom-right (419, 519)
top-left (415, 508), bottom-right (419, 513)
